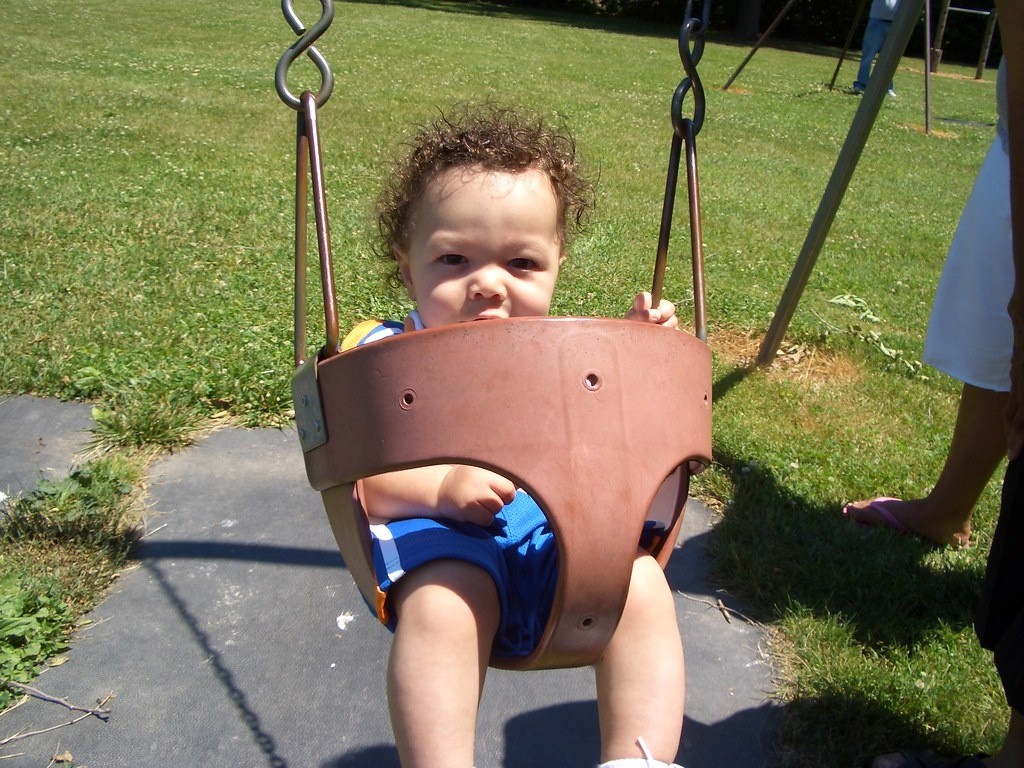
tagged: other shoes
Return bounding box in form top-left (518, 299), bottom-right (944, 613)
top-left (854, 87), bottom-right (862, 94)
top-left (887, 90), bottom-right (895, 97)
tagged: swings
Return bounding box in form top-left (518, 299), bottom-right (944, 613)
top-left (270, 0), bottom-right (714, 670)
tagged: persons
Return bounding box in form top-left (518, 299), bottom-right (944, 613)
top-left (341, 112), bottom-right (685, 768)
top-left (854, 0), bottom-right (898, 96)
top-left (845, 0), bottom-right (1023, 768)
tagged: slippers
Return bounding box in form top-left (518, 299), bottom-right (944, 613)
top-left (843, 496), bottom-right (969, 550)
